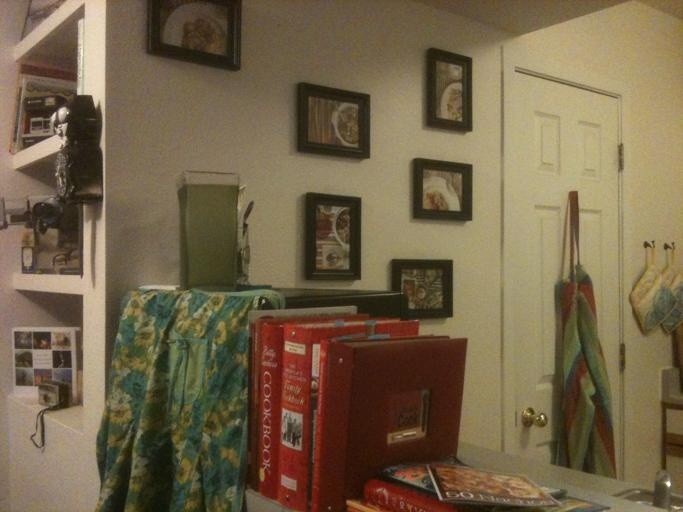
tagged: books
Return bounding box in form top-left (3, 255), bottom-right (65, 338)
top-left (243, 305), bottom-right (612, 511)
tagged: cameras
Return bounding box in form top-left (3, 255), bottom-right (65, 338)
top-left (22, 95), bottom-right (68, 148)
top-left (39, 379), bottom-right (71, 410)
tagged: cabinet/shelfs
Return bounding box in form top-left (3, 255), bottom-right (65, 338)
top-left (7, 0), bottom-right (84, 440)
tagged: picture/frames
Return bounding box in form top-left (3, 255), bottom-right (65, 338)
top-left (295, 81), bottom-right (373, 159)
top-left (302, 191), bottom-right (363, 281)
top-left (389, 257), bottom-right (455, 317)
top-left (144, 0), bottom-right (242, 72)
top-left (411, 156), bottom-right (474, 223)
top-left (426, 47), bottom-right (474, 132)
